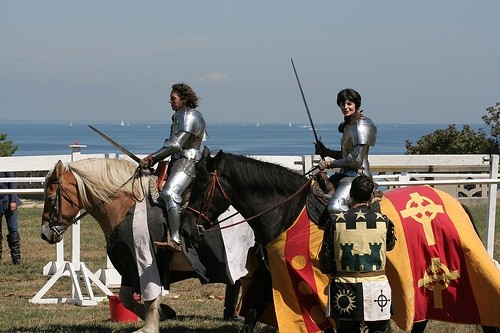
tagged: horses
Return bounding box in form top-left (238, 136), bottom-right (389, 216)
top-left (177, 144), bottom-right (499, 333)
top-left (39, 157), bottom-right (210, 333)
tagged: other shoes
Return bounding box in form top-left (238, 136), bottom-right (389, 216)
top-left (155, 238), bottom-right (182, 251)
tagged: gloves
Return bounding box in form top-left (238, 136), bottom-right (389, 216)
top-left (313, 141), bottom-right (327, 156)
top-left (139, 155), bottom-right (154, 170)
top-left (318, 160), bottom-right (332, 169)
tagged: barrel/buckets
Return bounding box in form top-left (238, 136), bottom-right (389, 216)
top-left (107, 294), bottom-right (140, 323)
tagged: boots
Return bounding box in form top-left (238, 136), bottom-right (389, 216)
top-left (6, 232), bottom-right (22, 265)
top-left (0, 236), bottom-right (3, 260)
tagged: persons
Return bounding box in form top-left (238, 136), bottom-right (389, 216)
top-left (139, 84), bottom-right (207, 246)
top-left (315, 89), bottom-right (377, 220)
top-left (0, 171), bottom-right (24, 266)
top-left (318, 175), bottom-right (397, 333)
